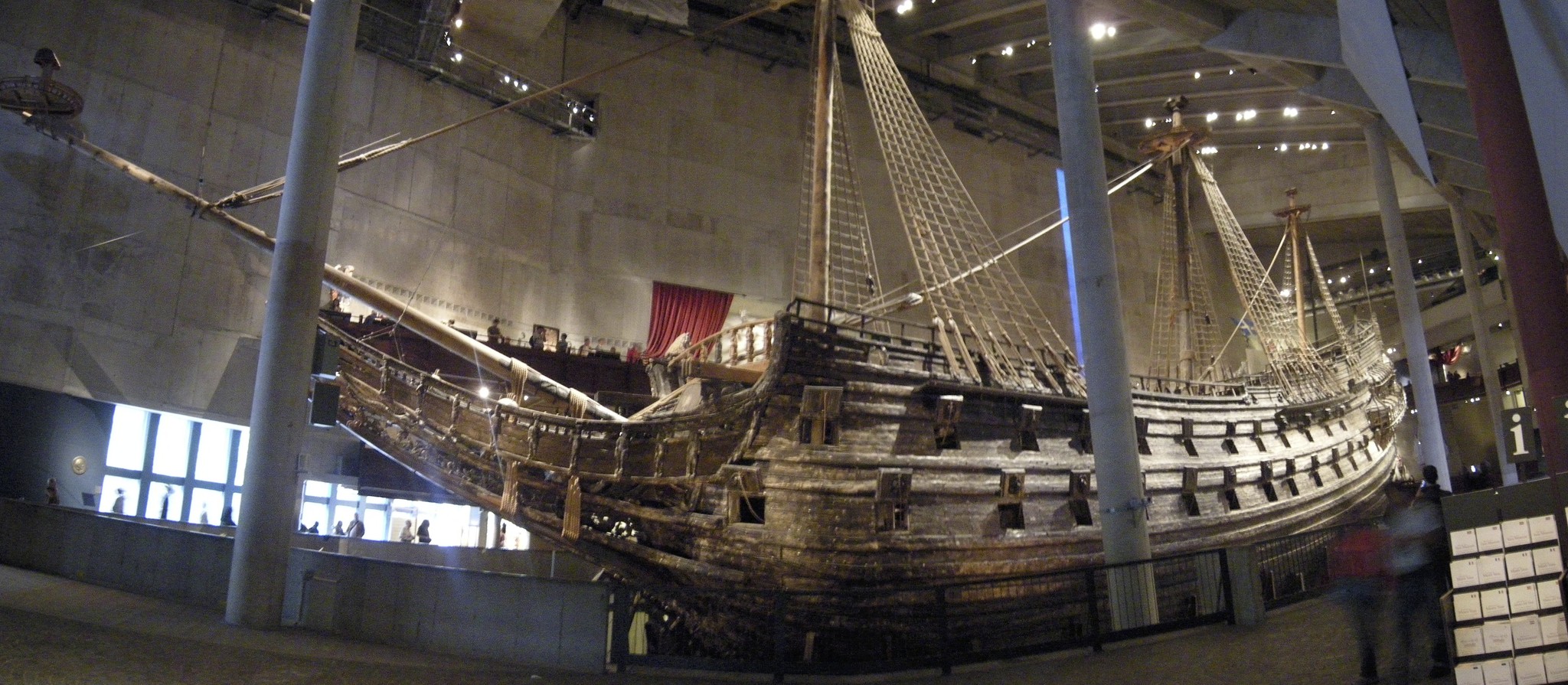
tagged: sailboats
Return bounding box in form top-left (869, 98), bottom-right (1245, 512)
top-left (309, 1), bottom-right (1414, 665)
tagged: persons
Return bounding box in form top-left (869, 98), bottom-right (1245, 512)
top-left (577, 337), bottom-right (616, 358)
top-left (399, 520), bottom-right (415, 543)
top-left (555, 333), bottom-right (568, 353)
top-left (345, 513), bottom-right (365, 537)
top-left (1407, 381), bottom-right (1411, 385)
top-left (113, 486), bottom-right (236, 526)
top-left (331, 521), bottom-right (345, 535)
top-left (500, 523), bottom-right (506, 547)
top-left (322, 265), bottom-right (382, 324)
top-left (416, 520), bottom-right (431, 545)
top-left (487, 318), bottom-right (511, 345)
top-left (301, 521), bottom-right (319, 533)
top-left (447, 319), bottom-right (454, 329)
top-left (528, 326), bottom-right (546, 351)
top-left (45, 477), bottom-right (59, 505)
top-left (1418, 465), bottom-right (1452, 498)
top-left (1446, 370), bottom-right (1470, 382)
